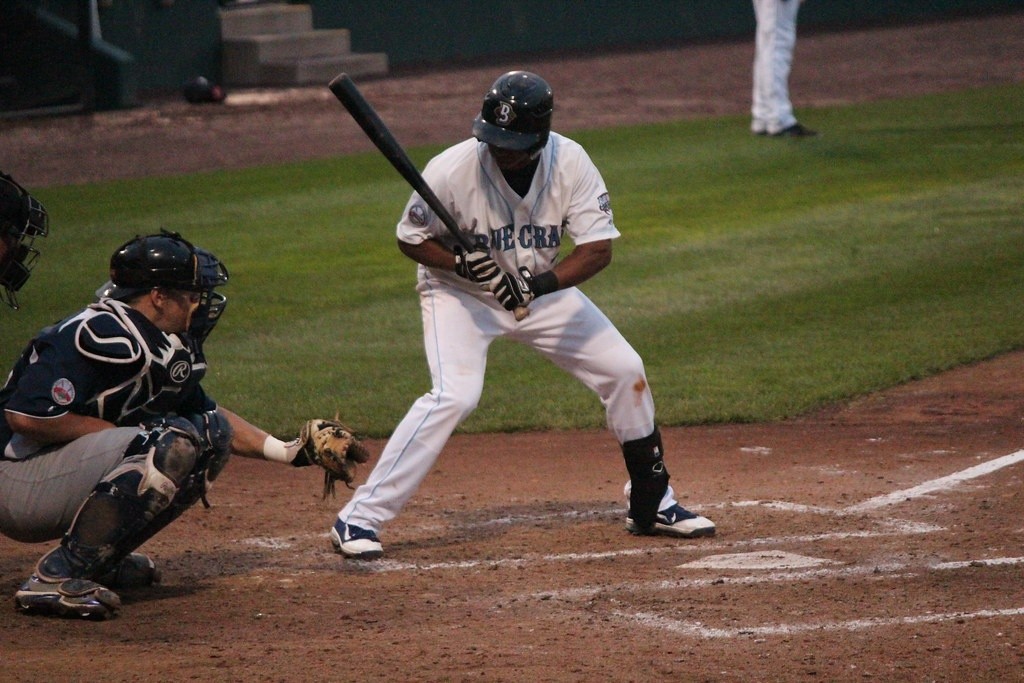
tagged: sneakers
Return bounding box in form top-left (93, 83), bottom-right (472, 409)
top-left (94, 551), bottom-right (157, 590)
top-left (625, 503), bottom-right (716, 539)
top-left (329, 516), bottom-right (383, 557)
top-left (14, 570), bottom-right (120, 620)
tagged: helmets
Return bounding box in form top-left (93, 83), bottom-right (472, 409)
top-left (0, 170), bottom-right (46, 236)
top-left (106, 227), bottom-right (218, 300)
top-left (472, 70), bottom-right (554, 150)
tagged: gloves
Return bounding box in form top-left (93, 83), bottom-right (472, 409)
top-left (490, 266), bottom-right (560, 311)
top-left (454, 243), bottom-right (501, 287)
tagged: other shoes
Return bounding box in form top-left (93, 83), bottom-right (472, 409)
top-left (755, 123), bottom-right (817, 137)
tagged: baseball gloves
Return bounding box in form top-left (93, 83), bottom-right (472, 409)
top-left (286, 415), bottom-right (370, 500)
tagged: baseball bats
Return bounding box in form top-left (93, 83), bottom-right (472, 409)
top-left (327, 74), bottom-right (532, 322)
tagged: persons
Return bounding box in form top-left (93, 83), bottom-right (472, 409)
top-left (330, 72), bottom-right (714, 558)
top-left (0, 169), bottom-right (52, 309)
top-left (0, 229), bottom-right (366, 619)
top-left (750, 0), bottom-right (819, 140)
